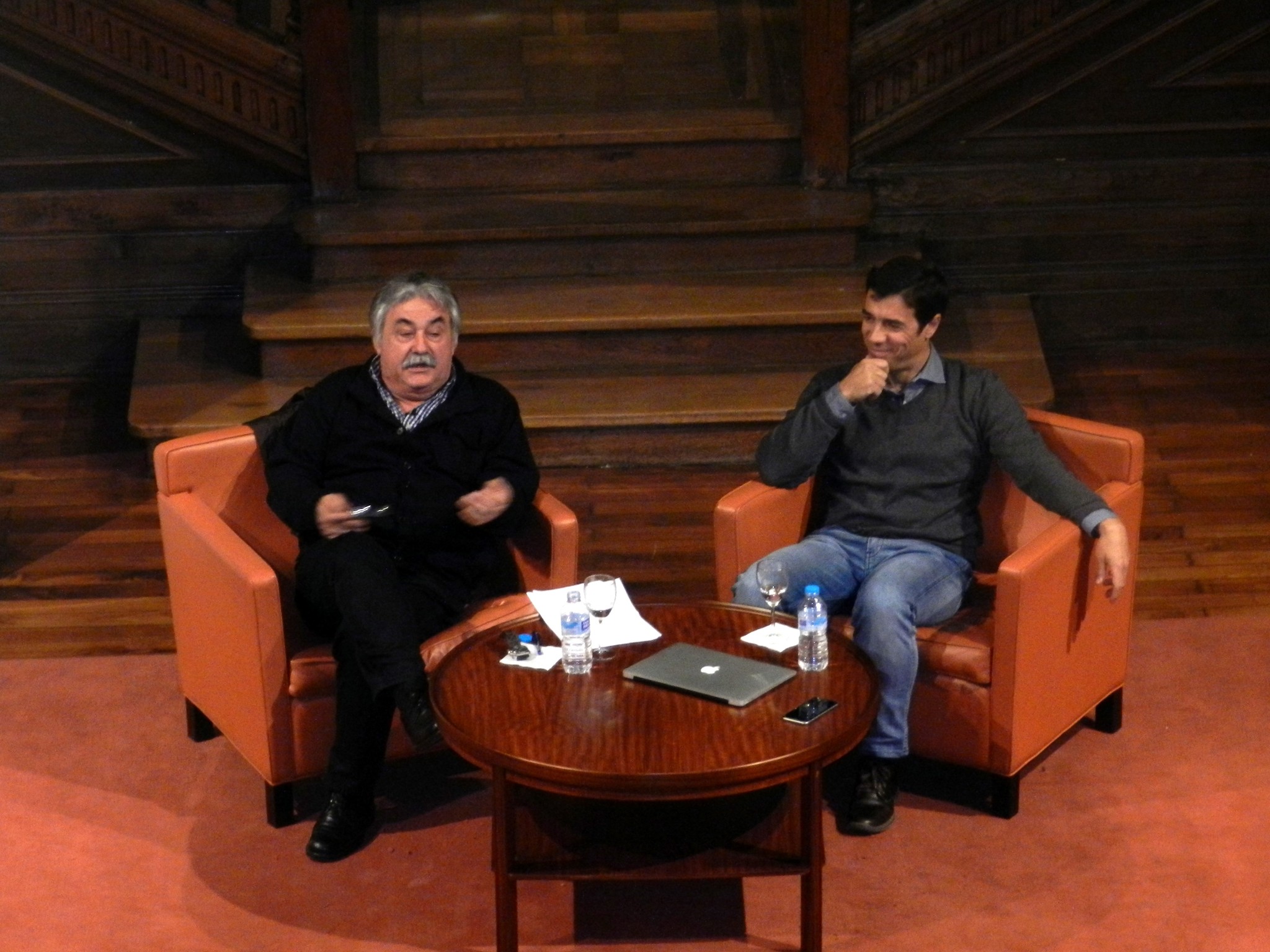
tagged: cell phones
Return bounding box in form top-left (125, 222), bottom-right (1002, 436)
top-left (782, 695), bottom-right (839, 725)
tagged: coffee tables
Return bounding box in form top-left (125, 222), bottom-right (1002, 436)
top-left (427, 593), bottom-right (883, 951)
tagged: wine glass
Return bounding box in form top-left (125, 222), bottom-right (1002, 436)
top-left (756, 559), bottom-right (789, 643)
top-left (584, 572), bottom-right (614, 661)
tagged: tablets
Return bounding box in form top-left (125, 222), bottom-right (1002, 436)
top-left (623, 640), bottom-right (798, 709)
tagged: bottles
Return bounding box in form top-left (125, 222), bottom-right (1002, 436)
top-left (798, 585), bottom-right (830, 672)
top-left (559, 592), bottom-right (595, 674)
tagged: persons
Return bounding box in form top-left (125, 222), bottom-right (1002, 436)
top-left (266, 271), bottom-right (540, 860)
top-left (724, 252), bottom-right (1134, 839)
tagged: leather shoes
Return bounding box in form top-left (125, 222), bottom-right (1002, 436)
top-left (398, 689), bottom-right (443, 752)
top-left (845, 756), bottom-right (899, 834)
top-left (305, 792), bottom-right (379, 863)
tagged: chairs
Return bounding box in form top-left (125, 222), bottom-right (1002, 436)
top-left (152, 426), bottom-right (579, 828)
top-left (711, 399), bottom-right (1149, 818)
